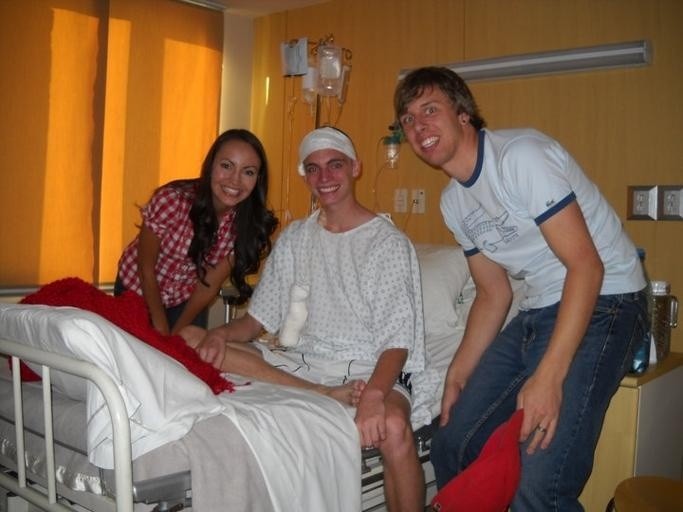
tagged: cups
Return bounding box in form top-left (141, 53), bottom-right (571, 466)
top-left (647, 280), bottom-right (679, 366)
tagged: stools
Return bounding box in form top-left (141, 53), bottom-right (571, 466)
top-left (605, 474), bottom-right (680, 512)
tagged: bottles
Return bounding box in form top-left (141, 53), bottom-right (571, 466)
top-left (314, 45), bottom-right (343, 98)
top-left (627, 247), bottom-right (653, 375)
top-left (301, 56), bottom-right (318, 104)
top-left (381, 134), bottom-right (401, 171)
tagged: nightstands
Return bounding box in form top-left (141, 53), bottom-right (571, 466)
top-left (579, 351), bottom-right (682, 512)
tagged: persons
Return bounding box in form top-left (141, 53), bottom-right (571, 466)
top-left (114, 128), bottom-right (276, 337)
top-left (180, 127), bottom-right (426, 511)
top-left (395, 66), bottom-right (651, 512)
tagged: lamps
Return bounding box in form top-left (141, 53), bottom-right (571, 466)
top-left (398, 40), bottom-right (652, 81)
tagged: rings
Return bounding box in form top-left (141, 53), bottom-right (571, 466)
top-left (538, 425), bottom-right (547, 434)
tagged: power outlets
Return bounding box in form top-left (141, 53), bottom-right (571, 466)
top-left (626, 185), bottom-right (657, 220)
top-left (411, 189), bottom-right (426, 215)
top-left (657, 184), bottom-right (682, 222)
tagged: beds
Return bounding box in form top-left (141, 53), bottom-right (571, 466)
top-left (0, 241), bottom-right (527, 512)
